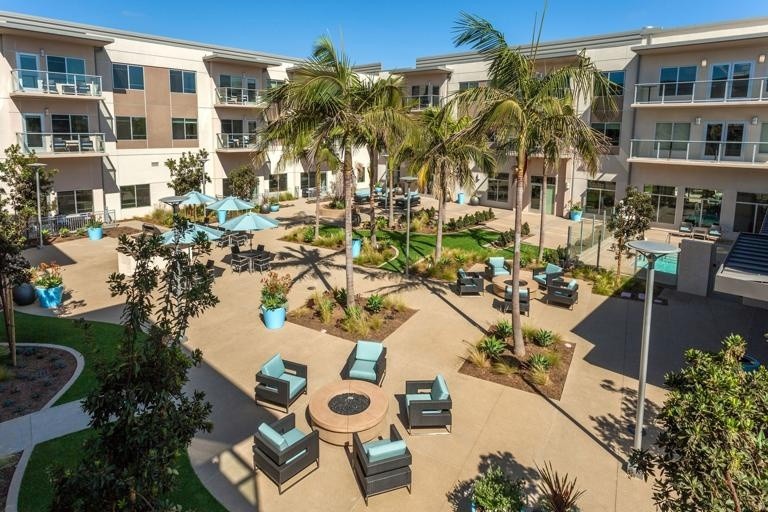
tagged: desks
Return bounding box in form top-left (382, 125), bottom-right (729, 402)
top-left (309, 378), bottom-right (389, 446)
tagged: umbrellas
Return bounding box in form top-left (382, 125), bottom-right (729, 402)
top-left (207, 194), bottom-right (257, 217)
top-left (220, 212), bottom-right (278, 252)
top-left (156, 220), bottom-right (225, 265)
top-left (181, 188), bottom-right (213, 222)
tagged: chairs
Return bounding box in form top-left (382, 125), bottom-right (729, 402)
top-left (43, 78), bottom-right (104, 96)
top-left (665, 216), bottom-right (728, 243)
top-left (115, 217), bottom-right (276, 293)
top-left (403, 373), bottom-right (452, 435)
top-left (453, 249), bottom-right (582, 318)
top-left (338, 339), bottom-right (388, 384)
top-left (253, 352), bottom-right (310, 412)
top-left (216, 134), bottom-right (252, 148)
top-left (252, 413), bottom-right (322, 493)
top-left (351, 422), bottom-right (414, 506)
top-left (215, 87), bottom-right (264, 105)
top-left (353, 182), bottom-right (419, 213)
top-left (49, 136), bottom-right (103, 152)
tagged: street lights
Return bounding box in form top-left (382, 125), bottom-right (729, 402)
top-left (158, 195), bottom-right (192, 344)
top-left (195, 156), bottom-right (210, 219)
top-left (380, 154), bottom-right (389, 215)
top-left (399, 176), bottom-right (420, 278)
top-left (27, 161), bottom-right (48, 248)
top-left (623, 240), bottom-right (682, 481)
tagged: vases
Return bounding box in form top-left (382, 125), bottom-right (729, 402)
top-left (263, 305), bottom-right (286, 330)
top-left (445, 189), bottom-right (451, 202)
top-left (470, 195), bottom-right (481, 205)
top-left (88, 228), bottom-right (104, 241)
top-left (352, 237), bottom-right (360, 259)
top-left (458, 193), bottom-right (464, 204)
top-left (36, 286), bottom-right (64, 309)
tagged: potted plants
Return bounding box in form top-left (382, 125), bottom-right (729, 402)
top-left (11, 265), bottom-right (36, 305)
top-left (320, 168), bottom-right (357, 219)
top-left (466, 458), bottom-right (586, 512)
top-left (569, 200), bottom-right (583, 220)
top-left (40, 217), bottom-right (223, 512)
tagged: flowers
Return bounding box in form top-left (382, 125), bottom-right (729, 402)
top-left (85, 212), bottom-right (103, 232)
top-left (33, 261), bottom-right (63, 289)
top-left (258, 269), bottom-right (292, 312)
